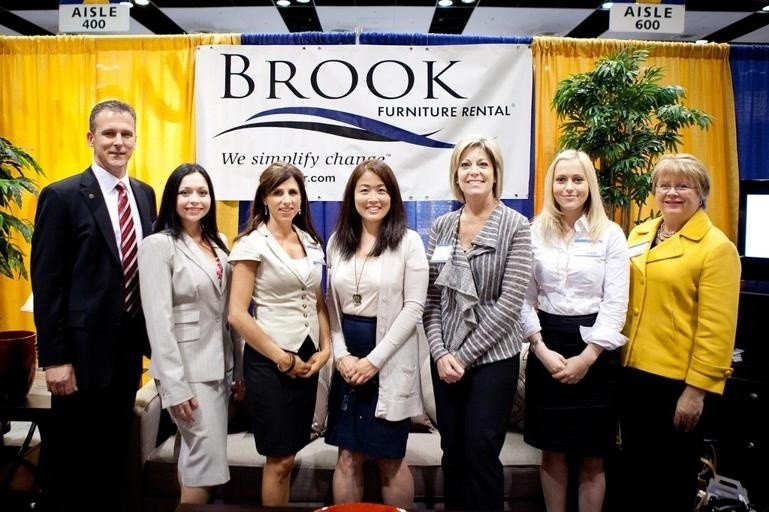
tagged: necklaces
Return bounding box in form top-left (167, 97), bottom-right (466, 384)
top-left (656, 222), bottom-right (676, 244)
top-left (350, 250), bottom-right (369, 307)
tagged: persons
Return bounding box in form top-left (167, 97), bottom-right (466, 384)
top-left (229, 165), bottom-right (333, 512)
top-left (420, 132), bottom-right (531, 512)
top-left (322, 159), bottom-right (432, 511)
top-left (517, 147), bottom-right (631, 512)
top-left (30, 98), bottom-right (160, 511)
top-left (620, 149), bottom-right (742, 510)
top-left (138, 160), bottom-right (246, 510)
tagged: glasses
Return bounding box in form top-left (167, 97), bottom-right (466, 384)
top-left (659, 183), bottom-right (694, 191)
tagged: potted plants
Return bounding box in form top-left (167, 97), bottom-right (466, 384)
top-left (0, 138), bottom-right (43, 407)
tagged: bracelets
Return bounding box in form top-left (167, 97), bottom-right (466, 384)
top-left (277, 351), bottom-right (296, 376)
top-left (529, 338), bottom-right (544, 353)
